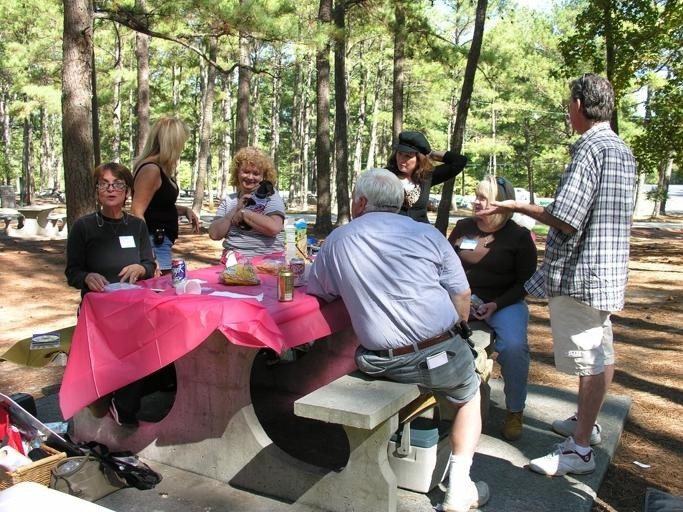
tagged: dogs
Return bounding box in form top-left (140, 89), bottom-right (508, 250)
top-left (238, 180), bottom-right (274, 231)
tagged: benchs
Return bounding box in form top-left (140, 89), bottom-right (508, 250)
top-left (294, 317), bottom-right (496, 512)
top-left (1, 325), bottom-right (112, 448)
top-left (0, 215), bottom-right (20, 228)
top-left (47, 215), bottom-right (67, 231)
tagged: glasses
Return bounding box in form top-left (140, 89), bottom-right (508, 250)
top-left (95, 182), bottom-right (128, 192)
top-left (496, 177), bottom-right (506, 193)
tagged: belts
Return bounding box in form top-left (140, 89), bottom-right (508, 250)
top-left (378, 327), bottom-right (458, 357)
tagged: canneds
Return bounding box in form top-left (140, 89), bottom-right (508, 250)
top-left (276, 268), bottom-right (294, 301)
top-left (288, 257), bottom-right (306, 287)
top-left (171, 259), bottom-right (186, 288)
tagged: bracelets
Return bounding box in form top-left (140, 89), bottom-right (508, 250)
top-left (240, 207), bottom-right (245, 222)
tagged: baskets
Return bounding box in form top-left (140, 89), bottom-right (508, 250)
top-left (0, 424), bottom-right (67, 490)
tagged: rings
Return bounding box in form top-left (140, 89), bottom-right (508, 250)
top-left (134, 277), bottom-right (137, 280)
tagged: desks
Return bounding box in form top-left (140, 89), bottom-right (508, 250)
top-left (18, 206), bottom-right (54, 235)
top-left (58, 250), bottom-right (345, 512)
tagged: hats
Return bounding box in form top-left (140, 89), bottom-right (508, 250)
top-left (392, 132), bottom-right (431, 154)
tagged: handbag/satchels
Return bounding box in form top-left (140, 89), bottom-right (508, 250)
top-left (50, 453), bottom-right (129, 502)
top-left (103, 450), bottom-right (161, 490)
top-left (220, 263), bottom-right (260, 285)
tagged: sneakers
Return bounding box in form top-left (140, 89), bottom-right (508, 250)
top-left (552, 412), bottom-right (602, 445)
top-left (504, 408), bottom-right (523, 440)
top-left (529, 436), bottom-right (596, 476)
top-left (109, 397), bottom-right (136, 427)
top-left (442, 481), bottom-right (490, 512)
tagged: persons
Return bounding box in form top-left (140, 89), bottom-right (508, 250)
top-left (209, 146), bottom-right (286, 264)
top-left (383, 131), bottom-right (466, 223)
top-left (65, 162), bottom-right (156, 428)
top-left (131, 118), bottom-right (200, 276)
top-left (448, 174), bottom-right (537, 441)
top-left (306, 169), bottom-right (490, 512)
top-left (476, 73), bottom-right (635, 477)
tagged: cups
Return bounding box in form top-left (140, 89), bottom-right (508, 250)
top-left (175, 280), bottom-right (202, 296)
